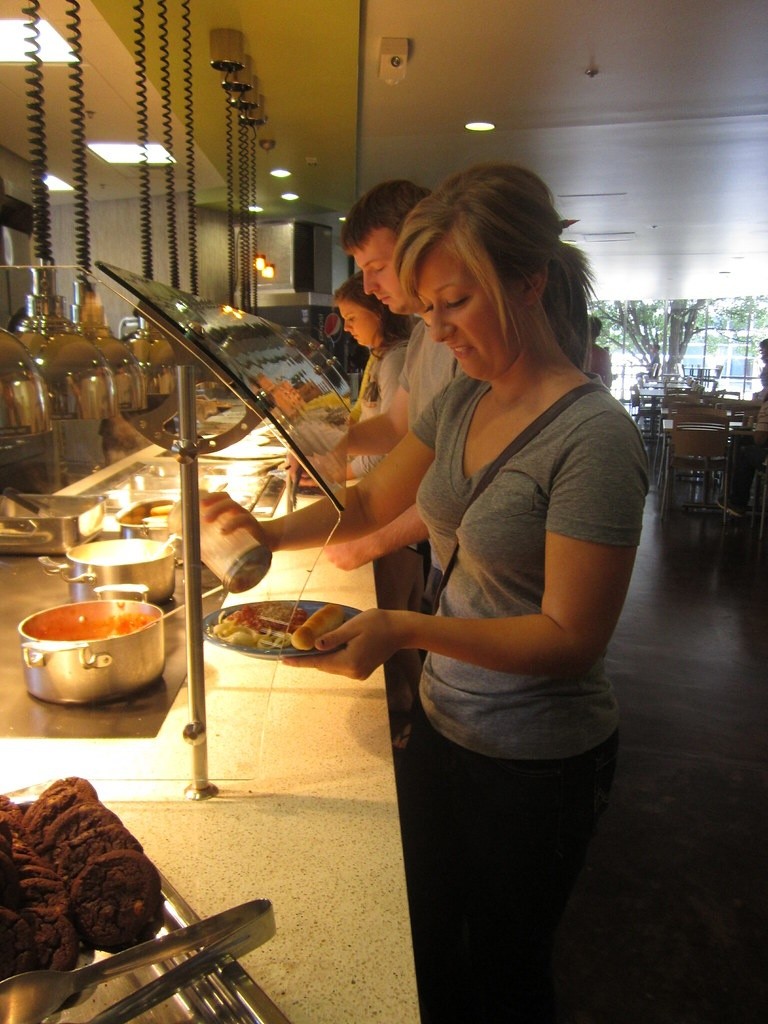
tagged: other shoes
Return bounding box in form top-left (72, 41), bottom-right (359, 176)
top-left (717, 495), bottom-right (745, 517)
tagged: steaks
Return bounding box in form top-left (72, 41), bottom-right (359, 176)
top-left (244, 601), bottom-right (308, 633)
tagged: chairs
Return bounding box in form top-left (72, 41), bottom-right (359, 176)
top-left (627, 362), bottom-right (768, 542)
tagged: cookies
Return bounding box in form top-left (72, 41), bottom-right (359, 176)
top-left (0, 776), bottom-right (166, 1010)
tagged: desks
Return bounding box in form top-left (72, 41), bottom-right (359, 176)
top-left (661, 408), bottom-right (745, 481)
top-left (662, 374), bottom-right (681, 381)
top-left (639, 389), bottom-right (688, 434)
top-left (643, 383), bottom-right (692, 388)
top-left (663, 419), bottom-right (758, 507)
top-left (660, 399), bottom-right (751, 407)
top-left (684, 367), bottom-right (718, 377)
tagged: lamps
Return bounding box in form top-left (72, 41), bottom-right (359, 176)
top-left (0, 1), bottom-right (265, 438)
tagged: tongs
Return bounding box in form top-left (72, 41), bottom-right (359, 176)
top-left (0, 898), bottom-right (276, 1024)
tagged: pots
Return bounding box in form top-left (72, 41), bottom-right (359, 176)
top-left (16, 584), bottom-right (165, 704)
top-left (38, 498), bottom-right (196, 605)
top-left (0, 488), bottom-right (108, 554)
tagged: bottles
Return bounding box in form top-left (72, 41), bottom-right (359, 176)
top-left (170, 491), bottom-right (272, 593)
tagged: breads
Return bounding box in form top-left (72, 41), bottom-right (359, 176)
top-left (291, 603), bottom-right (345, 651)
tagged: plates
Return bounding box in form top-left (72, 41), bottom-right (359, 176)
top-left (199, 600), bottom-right (367, 660)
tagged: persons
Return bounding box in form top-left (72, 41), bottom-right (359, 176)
top-left (191, 161), bottom-right (649, 1023)
top-left (717, 338), bottom-right (768, 518)
top-left (586, 310), bottom-right (612, 393)
top-left (325, 174), bottom-right (465, 858)
